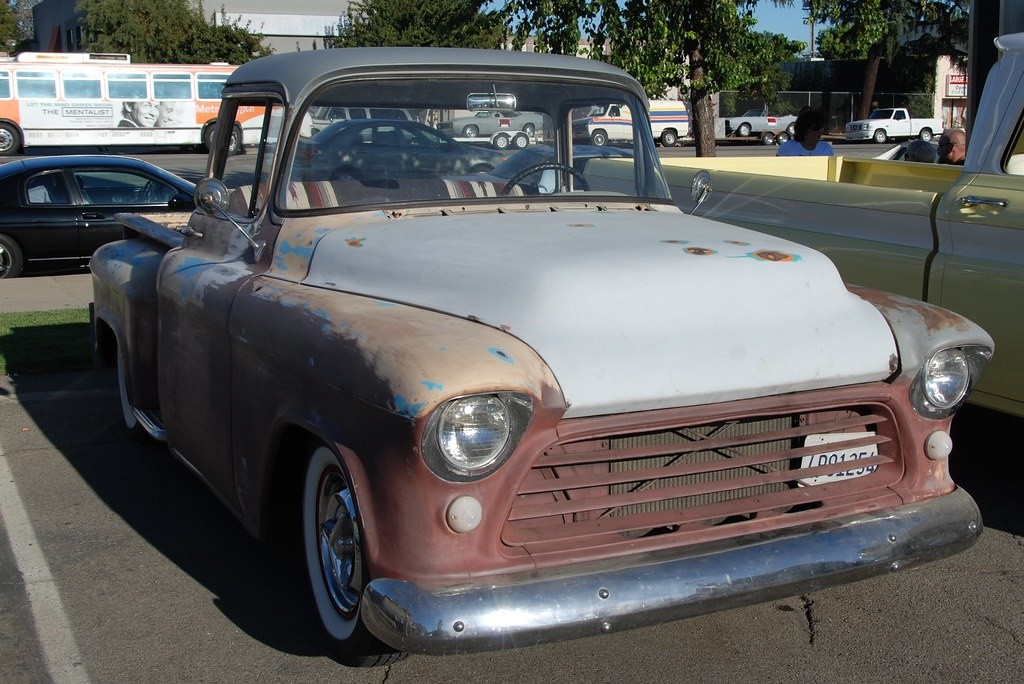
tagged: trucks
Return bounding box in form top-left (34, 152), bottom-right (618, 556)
top-left (571, 98), bottom-right (689, 148)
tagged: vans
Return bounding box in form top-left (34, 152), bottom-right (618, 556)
top-left (310, 105), bottom-right (414, 144)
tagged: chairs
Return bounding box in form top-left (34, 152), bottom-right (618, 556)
top-left (75, 174), bottom-right (93, 204)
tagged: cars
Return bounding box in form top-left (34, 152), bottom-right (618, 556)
top-left (293, 119), bottom-right (512, 182)
top-left (0, 152), bottom-right (200, 278)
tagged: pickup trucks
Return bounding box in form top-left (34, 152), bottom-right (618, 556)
top-left (582, 30), bottom-right (1024, 427)
top-left (845, 107), bottom-right (946, 145)
top-left (86, 48), bottom-right (997, 671)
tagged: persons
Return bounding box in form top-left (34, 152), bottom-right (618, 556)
top-left (905, 140), bottom-right (937, 163)
top-left (117, 101), bottom-right (175, 128)
top-left (776, 106), bottom-right (835, 156)
top-left (936, 129), bottom-right (966, 166)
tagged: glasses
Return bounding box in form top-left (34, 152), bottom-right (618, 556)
top-left (939, 142), bottom-right (957, 149)
top-left (904, 152), bottom-right (909, 159)
top-left (805, 122), bottom-right (824, 131)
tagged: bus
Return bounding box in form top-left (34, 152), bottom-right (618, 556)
top-left (0, 52), bottom-right (313, 158)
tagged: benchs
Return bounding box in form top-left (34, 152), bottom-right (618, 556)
top-left (227, 180), bottom-right (539, 220)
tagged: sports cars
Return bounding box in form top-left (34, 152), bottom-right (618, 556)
top-left (725, 107), bottom-right (798, 137)
top-left (434, 108), bottom-right (553, 139)
